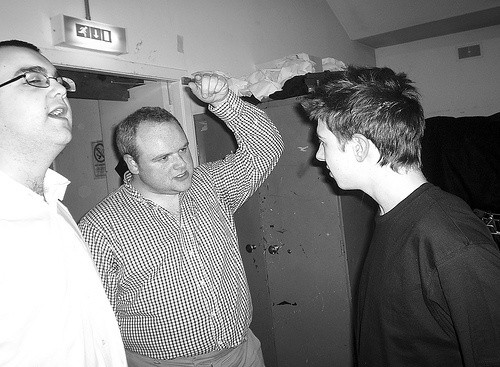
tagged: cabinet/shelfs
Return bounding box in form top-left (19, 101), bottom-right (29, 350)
top-left (194, 92), bottom-right (378, 367)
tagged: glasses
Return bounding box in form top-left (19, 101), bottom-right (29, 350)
top-left (0, 71), bottom-right (76, 92)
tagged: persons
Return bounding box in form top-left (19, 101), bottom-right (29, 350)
top-left (78, 72), bottom-right (285, 367)
top-left (301, 66), bottom-right (500, 367)
top-left (0, 39), bottom-right (128, 367)
